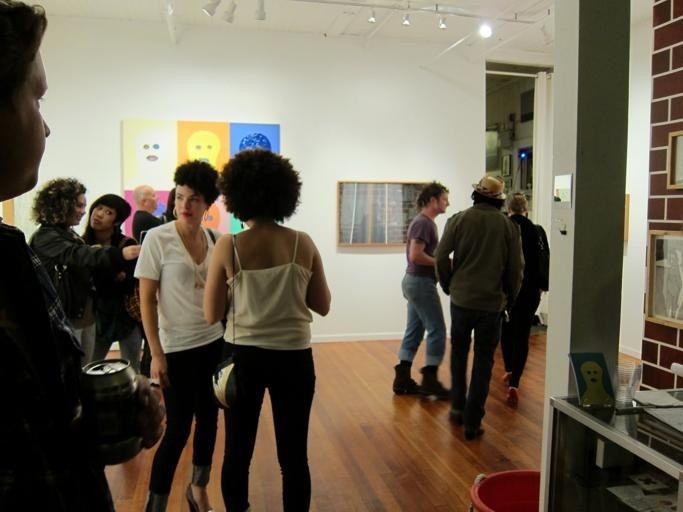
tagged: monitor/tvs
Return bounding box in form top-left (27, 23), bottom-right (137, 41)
top-left (486, 127), bottom-right (500, 172)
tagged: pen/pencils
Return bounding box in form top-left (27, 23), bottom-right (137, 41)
top-left (616, 406), bottom-right (656, 413)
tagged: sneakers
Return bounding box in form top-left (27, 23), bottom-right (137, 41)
top-left (507, 387), bottom-right (519, 407)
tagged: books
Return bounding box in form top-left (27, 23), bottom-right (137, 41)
top-left (634, 387), bottom-right (683, 451)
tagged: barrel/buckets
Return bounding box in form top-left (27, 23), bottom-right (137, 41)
top-left (467, 467), bottom-right (541, 512)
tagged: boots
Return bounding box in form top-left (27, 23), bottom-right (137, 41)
top-left (417, 365), bottom-right (451, 399)
top-left (393, 363), bottom-right (422, 394)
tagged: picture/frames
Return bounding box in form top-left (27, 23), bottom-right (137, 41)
top-left (665, 129), bottom-right (683, 190)
top-left (642, 230), bottom-right (683, 331)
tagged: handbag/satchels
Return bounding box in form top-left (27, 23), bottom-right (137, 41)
top-left (212, 357), bottom-right (238, 409)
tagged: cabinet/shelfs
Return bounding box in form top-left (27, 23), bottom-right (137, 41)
top-left (538, 387), bottom-right (683, 512)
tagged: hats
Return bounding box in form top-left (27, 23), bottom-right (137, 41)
top-left (472, 175), bottom-right (507, 199)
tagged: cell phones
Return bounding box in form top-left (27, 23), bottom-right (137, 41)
top-left (140, 231), bottom-right (148, 245)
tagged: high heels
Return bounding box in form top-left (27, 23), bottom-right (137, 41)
top-left (185, 483), bottom-right (214, 511)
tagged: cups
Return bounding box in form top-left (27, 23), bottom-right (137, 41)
top-left (609, 403), bottom-right (637, 440)
top-left (611, 359), bottom-right (642, 400)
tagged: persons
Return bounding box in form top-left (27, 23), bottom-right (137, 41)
top-left (500, 190), bottom-right (550, 407)
top-left (433, 175), bottom-right (526, 439)
top-left (135, 159), bottom-right (229, 511)
top-left (30, 177), bottom-right (175, 381)
top-left (0, 0), bottom-right (165, 510)
top-left (393, 182), bottom-right (451, 400)
top-left (202, 147), bottom-right (331, 510)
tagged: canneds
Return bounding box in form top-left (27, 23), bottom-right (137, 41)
top-left (77, 358), bottom-right (145, 465)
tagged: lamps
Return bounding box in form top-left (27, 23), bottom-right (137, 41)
top-left (200, 2), bottom-right (451, 31)
